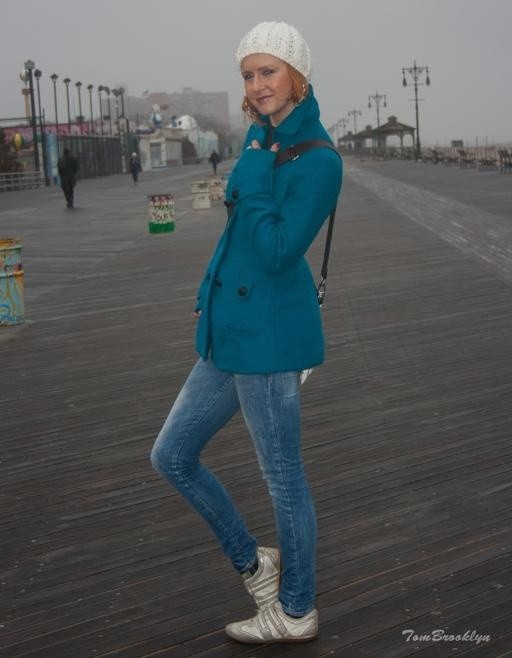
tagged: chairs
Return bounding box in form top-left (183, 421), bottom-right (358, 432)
top-left (390, 144), bottom-right (512, 175)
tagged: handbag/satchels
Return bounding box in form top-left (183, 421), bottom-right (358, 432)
top-left (297, 289), bottom-right (325, 387)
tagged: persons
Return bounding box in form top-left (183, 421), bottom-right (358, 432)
top-left (57, 148), bottom-right (80, 208)
top-left (128, 151), bottom-right (143, 183)
top-left (148, 19), bottom-right (344, 648)
top-left (208, 149), bottom-right (220, 176)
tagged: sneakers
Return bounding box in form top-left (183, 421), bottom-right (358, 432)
top-left (240, 547), bottom-right (282, 608)
top-left (224, 602), bottom-right (320, 644)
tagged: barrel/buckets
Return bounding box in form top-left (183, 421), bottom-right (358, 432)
top-left (147, 194), bottom-right (175, 233)
top-left (191, 179), bottom-right (227, 210)
top-left (0, 237), bottom-right (26, 327)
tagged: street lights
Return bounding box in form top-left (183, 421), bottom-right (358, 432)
top-left (326, 117), bottom-right (348, 148)
top-left (402, 59), bottom-right (431, 160)
top-left (20, 58), bottom-right (126, 186)
top-left (368, 89), bottom-right (387, 157)
top-left (347, 106), bottom-right (360, 157)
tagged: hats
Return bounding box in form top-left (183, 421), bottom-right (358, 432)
top-left (236, 21), bottom-right (312, 85)
